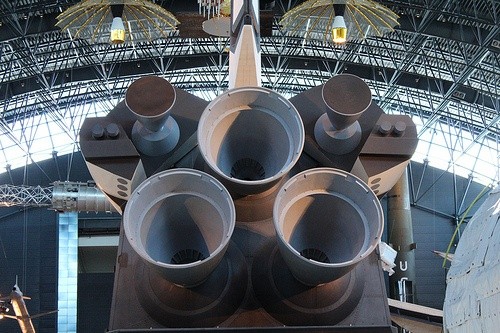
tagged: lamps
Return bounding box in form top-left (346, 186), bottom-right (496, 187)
top-left (278, 0), bottom-right (401, 43)
top-left (54, 0), bottom-right (181, 45)
top-left (197, 0), bottom-right (231, 37)
